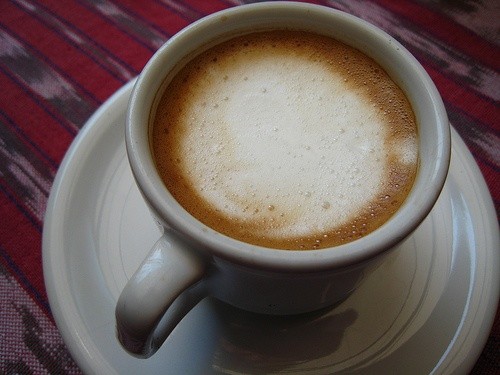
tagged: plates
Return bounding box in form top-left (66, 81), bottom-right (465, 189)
top-left (42, 74), bottom-right (500, 375)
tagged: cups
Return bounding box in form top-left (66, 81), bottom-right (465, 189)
top-left (109, 1), bottom-right (453, 359)
